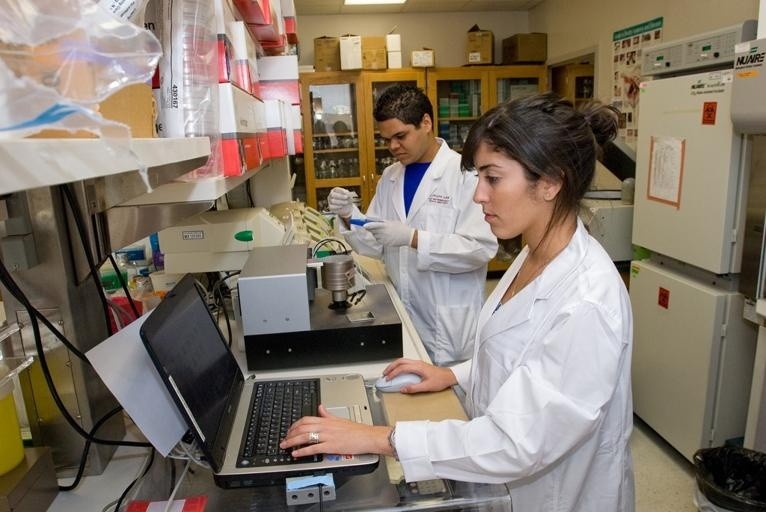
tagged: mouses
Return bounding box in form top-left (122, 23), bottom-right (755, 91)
top-left (377, 370), bottom-right (422, 396)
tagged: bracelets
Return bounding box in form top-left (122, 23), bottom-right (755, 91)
top-left (389, 426), bottom-right (400, 462)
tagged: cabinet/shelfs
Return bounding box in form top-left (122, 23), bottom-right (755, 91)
top-left (298, 65), bottom-right (550, 215)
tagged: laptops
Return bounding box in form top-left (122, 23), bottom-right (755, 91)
top-left (140, 267), bottom-right (380, 489)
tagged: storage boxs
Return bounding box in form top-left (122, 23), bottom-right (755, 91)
top-left (464, 22), bottom-right (547, 65)
top-left (411, 46), bottom-right (435, 68)
top-left (313, 24), bottom-right (403, 71)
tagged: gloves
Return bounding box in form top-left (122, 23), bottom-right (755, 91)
top-left (362, 215), bottom-right (416, 248)
top-left (326, 185), bottom-right (355, 220)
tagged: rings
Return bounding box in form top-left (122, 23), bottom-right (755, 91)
top-left (308, 431), bottom-right (320, 445)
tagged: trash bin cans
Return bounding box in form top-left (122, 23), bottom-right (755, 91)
top-left (693, 446), bottom-right (766, 512)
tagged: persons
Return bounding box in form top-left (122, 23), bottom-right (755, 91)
top-left (277, 88), bottom-right (638, 512)
top-left (326, 81), bottom-right (500, 367)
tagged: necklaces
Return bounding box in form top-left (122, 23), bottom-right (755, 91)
top-left (509, 253), bottom-right (553, 297)
